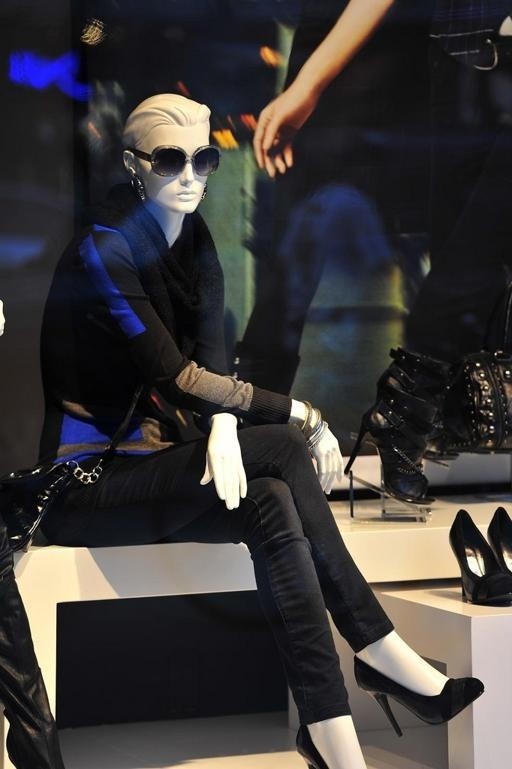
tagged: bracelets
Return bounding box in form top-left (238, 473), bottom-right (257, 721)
top-left (298, 400), bottom-right (328, 450)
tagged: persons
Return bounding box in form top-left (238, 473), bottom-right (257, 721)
top-left (37, 93), bottom-right (485, 768)
top-left (234, 0), bottom-right (512, 427)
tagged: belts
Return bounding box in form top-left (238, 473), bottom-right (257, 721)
top-left (427, 36), bottom-right (512, 74)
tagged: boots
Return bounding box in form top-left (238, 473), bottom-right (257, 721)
top-left (343, 347), bottom-right (452, 506)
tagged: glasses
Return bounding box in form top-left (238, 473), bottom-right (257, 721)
top-left (125, 145), bottom-right (222, 178)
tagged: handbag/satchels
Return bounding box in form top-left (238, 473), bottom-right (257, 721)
top-left (445, 350), bottom-right (511, 454)
top-left (4, 460), bottom-right (77, 546)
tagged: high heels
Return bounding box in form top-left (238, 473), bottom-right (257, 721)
top-left (295, 722), bottom-right (329, 769)
top-left (448, 509), bottom-right (512, 605)
top-left (485, 505), bottom-right (512, 573)
top-left (353, 652), bottom-right (486, 739)
